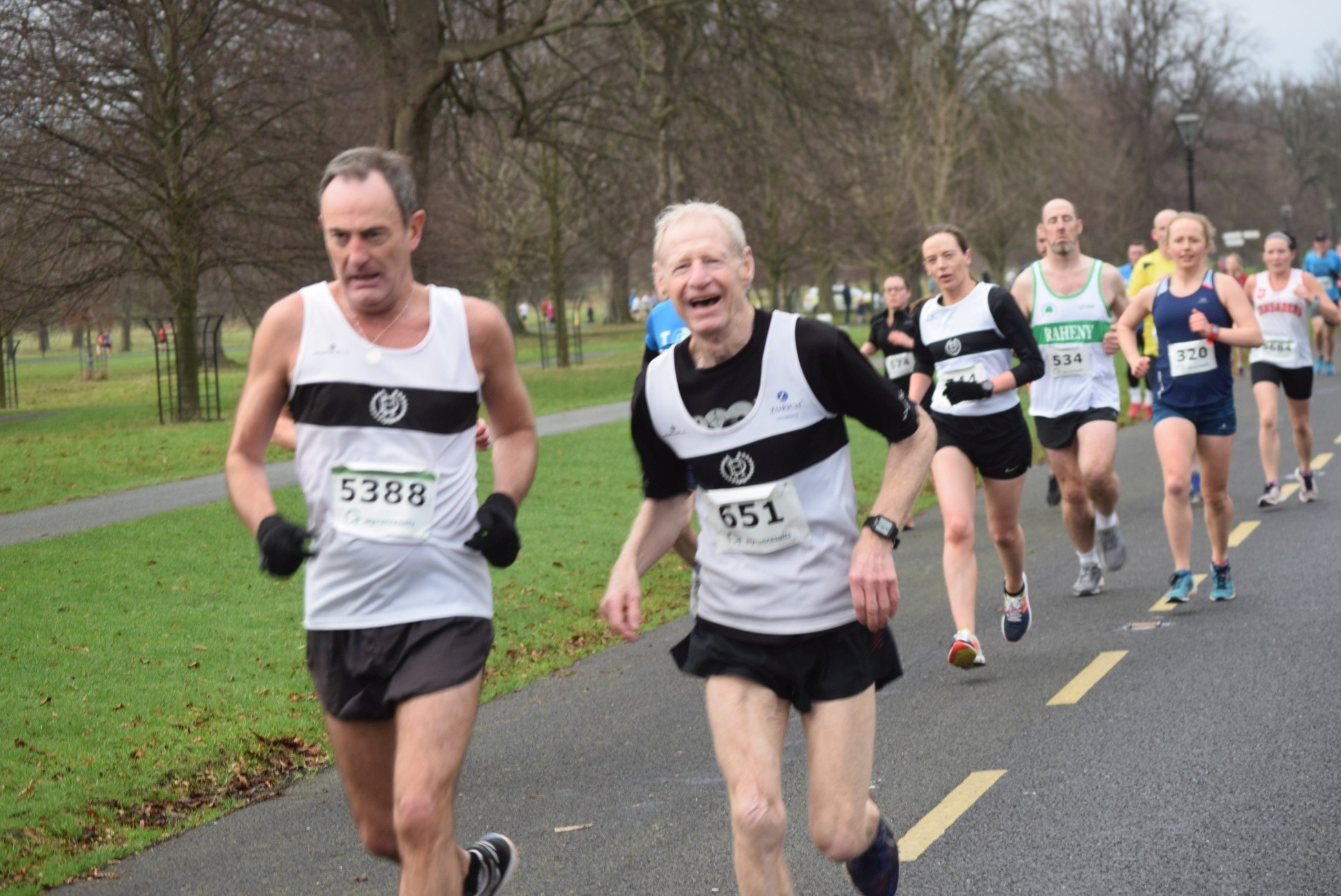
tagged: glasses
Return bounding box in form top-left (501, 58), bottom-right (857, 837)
top-left (885, 285), bottom-right (906, 292)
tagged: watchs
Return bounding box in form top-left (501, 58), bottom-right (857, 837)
top-left (982, 380), bottom-right (994, 398)
top-left (862, 514), bottom-right (900, 550)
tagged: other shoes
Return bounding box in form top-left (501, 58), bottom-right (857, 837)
top-left (1046, 474), bottom-right (1060, 506)
top-left (1315, 356), bottom-right (1323, 372)
top-left (904, 517), bottom-right (914, 529)
top-left (1127, 402), bottom-right (1141, 420)
top-left (1325, 360), bottom-right (1334, 375)
top-left (1143, 403), bottom-right (1153, 420)
top-left (1189, 472), bottom-right (1202, 505)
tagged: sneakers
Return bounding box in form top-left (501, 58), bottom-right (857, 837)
top-left (1166, 569), bottom-right (1198, 603)
top-left (1073, 562), bottom-right (1104, 596)
top-left (1209, 558), bottom-right (1236, 601)
top-left (464, 832), bottom-right (517, 896)
top-left (1295, 469), bottom-right (1318, 503)
top-left (947, 630), bottom-right (986, 669)
top-left (1096, 512), bottom-right (1125, 570)
top-left (847, 819), bottom-right (899, 896)
top-left (1258, 480), bottom-right (1281, 507)
top-left (1001, 571), bottom-right (1032, 642)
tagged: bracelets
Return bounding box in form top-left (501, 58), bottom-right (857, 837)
top-left (1312, 294), bottom-right (1320, 309)
top-left (1206, 323), bottom-right (1218, 345)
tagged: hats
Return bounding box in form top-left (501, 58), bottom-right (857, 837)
top-left (1315, 234), bottom-right (1327, 242)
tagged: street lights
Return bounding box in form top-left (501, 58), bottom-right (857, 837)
top-left (1171, 91), bottom-right (1200, 209)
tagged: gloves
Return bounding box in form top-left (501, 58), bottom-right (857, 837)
top-left (464, 493), bottom-right (521, 567)
top-left (943, 381), bottom-right (984, 405)
top-left (257, 515), bottom-right (311, 577)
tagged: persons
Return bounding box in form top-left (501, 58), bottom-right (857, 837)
top-left (1014, 223), bottom-right (1341, 509)
top-left (1010, 199), bottom-right (1129, 594)
top-left (224, 145), bottom-right (539, 896)
top-left (542, 298), bottom-right (594, 324)
top-left (906, 224), bottom-right (1046, 669)
top-left (157, 326), bottom-right (165, 351)
top-left (1114, 212), bottom-right (1264, 604)
top-left (841, 271), bottom-right (993, 530)
top-left (595, 198), bottom-right (938, 896)
top-left (96, 326), bottom-right (112, 358)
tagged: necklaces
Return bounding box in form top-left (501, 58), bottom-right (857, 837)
top-left (351, 283), bottom-right (415, 363)
